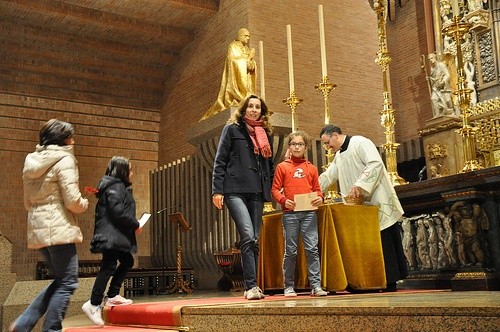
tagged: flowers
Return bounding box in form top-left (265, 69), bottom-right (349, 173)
top-left (83, 185), bottom-right (97, 200)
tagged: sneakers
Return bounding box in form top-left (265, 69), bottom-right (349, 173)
top-left (310, 287), bottom-right (327, 296)
top-left (81, 300), bottom-right (104, 327)
top-left (246, 286), bottom-right (262, 300)
top-left (284, 287), bottom-right (297, 297)
top-left (106, 294), bottom-right (133, 307)
top-left (244, 286), bottom-right (266, 299)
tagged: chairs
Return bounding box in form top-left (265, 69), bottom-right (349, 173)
top-left (213, 243), bottom-right (246, 289)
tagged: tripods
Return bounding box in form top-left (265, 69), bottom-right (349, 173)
top-left (168, 213), bottom-right (194, 293)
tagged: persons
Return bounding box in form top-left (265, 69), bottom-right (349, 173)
top-left (81, 157), bottom-right (142, 329)
top-left (212, 95), bottom-right (275, 300)
top-left (196, 28), bottom-right (257, 123)
top-left (271, 131), bottom-right (329, 297)
top-left (398, 202), bottom-right (488, 268)
top-left (424, 53), bottom-right (450, 118)
top-left (319, 125), bottom-right (409, 292)
top-left (8, 119), bottom-right (89, 332)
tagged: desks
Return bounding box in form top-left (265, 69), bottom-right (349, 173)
top-left (258, 201), bottom-right (384, 294)
top-left (124, 268), bottom-right (196, 298)
top-left (393, 165), bottom-right (500, 292)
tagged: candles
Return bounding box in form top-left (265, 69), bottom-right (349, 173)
top-left (286, 24), bottom-right (296, 97)
top-left (452, 0), bottom-right (460, 17)
top-left (318, 4), bottom-right (327, 83)
top-left (258, 39), bottom-right (266, 101)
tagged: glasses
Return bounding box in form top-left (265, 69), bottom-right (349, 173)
top-left (288, 141), bottom-right (306, 148)
top-left (325, 134), bottom-right (333, 146)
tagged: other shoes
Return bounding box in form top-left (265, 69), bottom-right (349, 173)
top-left (379, 283), bottom-right (397, 292)
